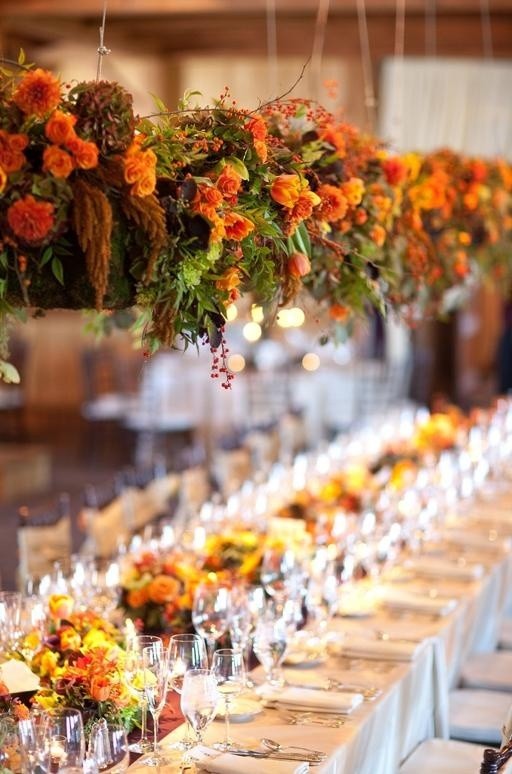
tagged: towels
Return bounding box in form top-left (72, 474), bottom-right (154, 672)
top-left (335, 638), bottom-right (425, 665)
top-left (262, 686), bottom-right (362, 715)
top-left (388, 592), bottom-right (460, 620)
top-left (207, 752), bottom-right (310, 774)
top-left (415, 561), bottom-right (481, 583)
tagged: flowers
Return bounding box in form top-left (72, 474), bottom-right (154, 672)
top-left (3, 65), bottom-right (510, 390)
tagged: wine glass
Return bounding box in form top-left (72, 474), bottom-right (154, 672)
top-left (0, 398), bottom-right (512, 774)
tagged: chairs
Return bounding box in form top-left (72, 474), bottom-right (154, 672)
top-left (12, 408), bottom-right (308, 586)
